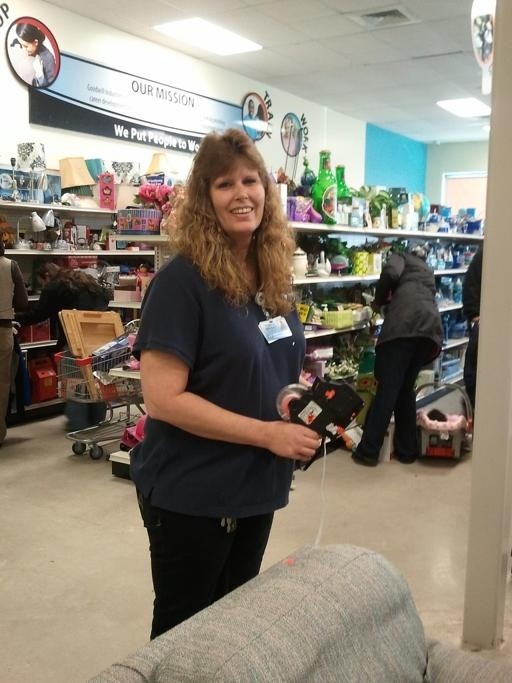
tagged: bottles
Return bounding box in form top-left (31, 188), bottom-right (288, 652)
top-left (310, 147), bottom-right (353, 227)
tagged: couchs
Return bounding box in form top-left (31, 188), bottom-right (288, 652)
top-left (84, 543), bottom-right (511, 682)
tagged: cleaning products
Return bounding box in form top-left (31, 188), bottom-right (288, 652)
top-left (426, 238), bottom-right (476, 308)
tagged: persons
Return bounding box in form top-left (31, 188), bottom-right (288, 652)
top-left (460, 244), bottom-right (484, 429)
top-left (15, 21), bottom-right (55, 89)
top-left (243, 99), bottom-right (262, 140)
top-left (20, 259), bottom-right (109, 433)
top-left (281, 116), bottom-right (296, 156)
top-left (0, 238), bottom-right (28, 449)
top-left (348, 249), bottom-right (444, 467)
top-left (129, 128), bottom-right (326, 641)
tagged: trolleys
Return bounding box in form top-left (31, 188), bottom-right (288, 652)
top-left (54, 317), bottom-right (147, 460)
